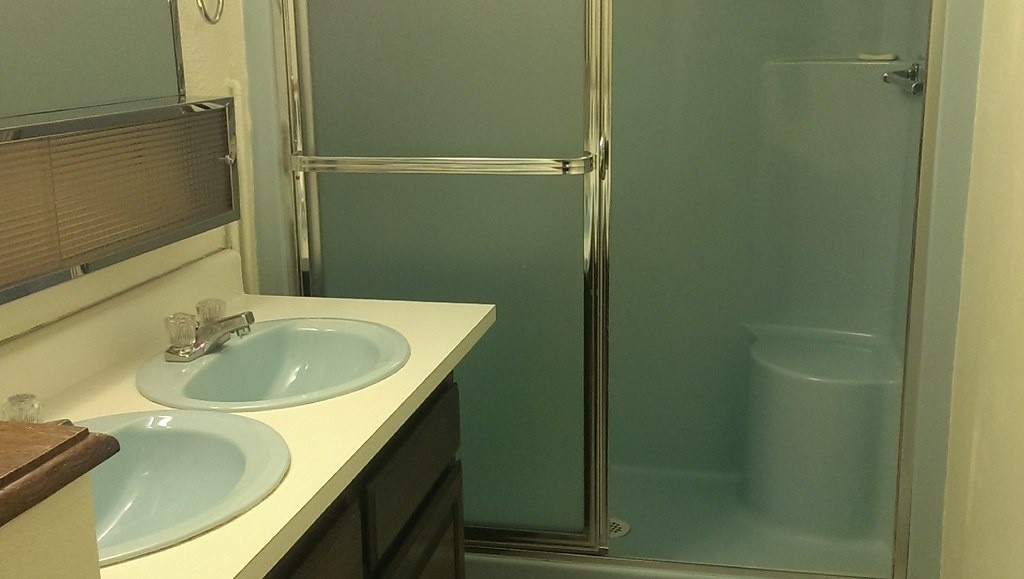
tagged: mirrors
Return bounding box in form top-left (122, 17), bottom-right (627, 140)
top-left (0, 0), bottom-right (186, 130)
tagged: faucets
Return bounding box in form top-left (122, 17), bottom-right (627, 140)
top-left (39, 419), bottom-right (75, 426)
top-left (196, 311), bottom-right (256, 356)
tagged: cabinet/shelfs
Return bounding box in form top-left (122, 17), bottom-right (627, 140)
top-left (260, 372), bottom-right (465, 579)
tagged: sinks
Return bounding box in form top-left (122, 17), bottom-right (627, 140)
top-left (1, 411), bottom-right (293, 568)
top-left (133, 319), bottom-right (413, 411)
